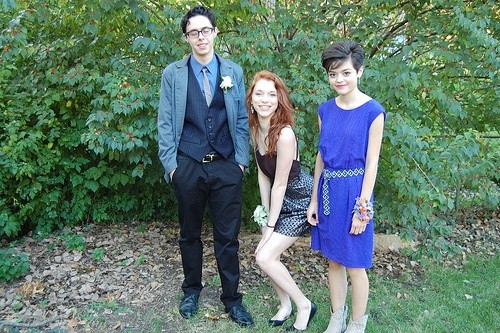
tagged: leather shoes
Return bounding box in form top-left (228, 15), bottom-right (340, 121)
top-left (178, 293), bottom-right (199, 319)
top-left (225, 304), bottom-right (254, 328)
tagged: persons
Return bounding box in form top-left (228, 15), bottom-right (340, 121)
top-left (157, 5), bottom-right (254, 327)
top-left (306, 39), bottom-right (387, 333)
top-left (244, 70), bottom-right (318, 333)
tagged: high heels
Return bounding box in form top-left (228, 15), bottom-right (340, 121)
top-left (268, 307), bottom-right (294, 328)
top-left (285, 301), bottom-right (317, 333)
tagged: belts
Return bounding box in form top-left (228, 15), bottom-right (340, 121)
top-left (322, 168), bottom-right (364, 216)
top-left (202, 153), bottom-right (222, 163)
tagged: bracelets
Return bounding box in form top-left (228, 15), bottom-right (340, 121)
top-left (350, 197), bottom-right (376, 223)
top-left (251, 205), bottom-right (268, 229)
top-left (266, 224), bottom-right (276, 228)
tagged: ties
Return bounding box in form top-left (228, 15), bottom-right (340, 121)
top-left (201, 68), bottom-right (212, 108)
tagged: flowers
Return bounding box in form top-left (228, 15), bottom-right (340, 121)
top-left (220, 76), bottom-right (233, 93)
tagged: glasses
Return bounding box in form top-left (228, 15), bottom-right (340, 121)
top-left (185, 26), bottom-right (215, 39)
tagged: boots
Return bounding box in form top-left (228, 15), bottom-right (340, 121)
top-left (343, 314), bottom-right (368, 333)
top-left (323, 305), bottom-right (348, 333)
